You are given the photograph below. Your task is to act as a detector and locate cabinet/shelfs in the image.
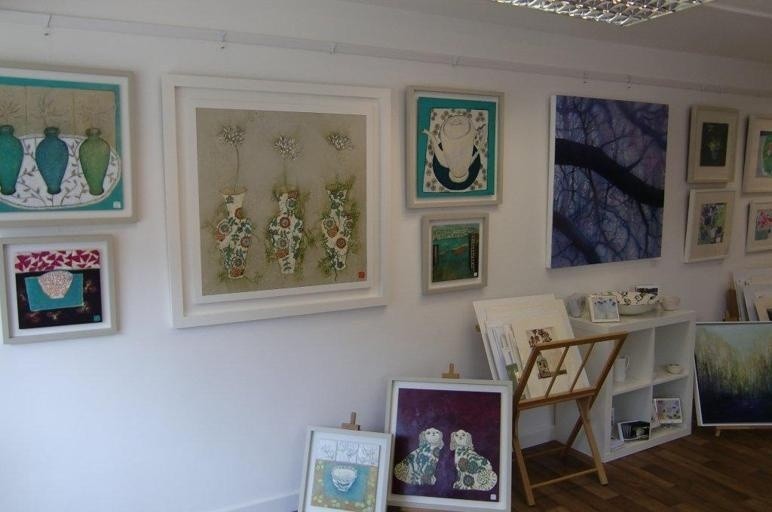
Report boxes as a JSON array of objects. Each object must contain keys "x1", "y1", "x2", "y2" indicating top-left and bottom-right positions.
[{"x1": 554, "y1": 310, "x2": 696, "y2": 463}]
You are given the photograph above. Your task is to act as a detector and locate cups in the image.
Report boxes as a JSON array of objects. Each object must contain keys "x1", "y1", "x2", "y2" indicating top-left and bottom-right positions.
[{"x1": 613, "y1": 354, "x2": 629, "y2": 382}]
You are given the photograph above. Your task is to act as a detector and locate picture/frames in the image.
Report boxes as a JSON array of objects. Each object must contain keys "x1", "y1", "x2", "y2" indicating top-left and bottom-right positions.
[
  {"x1": 420, "y1": 210, "x2": 489, "y2": 295},
  {"x1": 742, "y1": 113, "x2": 772, "y2": 194},
  {"x1": 694, "y1": 320, "x2": 772, "y2": 427},
  {"x1": 0, "y1": 65, "x2": 136, "y2": 226},
  {"x1": 683, "y1": 187, "x2": 737, "y2": 263},
  {"x1": 744, "y1": 198, "x2": 772, "y2": 254},
  {"x1": 297, "y1": 425, "x2": 394, "y2": 512},
  {"x1": 383, "y1": 376, "x2": 513, "y2": 512},
  {"x1": 588, "y1": 294, "x2": 620, "y2": 323},
  {"x1": 160, "y1": 72, "x2": 388, "y2": 328},
  {"x1": 405, "y1": 83, "x2": 506, "y2": 209},
  {"x1": 686, "y1": 102, "x2": 740, "y2": 185},
  {"x1": 0, "y1": 233, "x2": 119, "y2": 344},
  {"x1": 546, "y1": 90, "x2": 670, "y2": 267}
]
